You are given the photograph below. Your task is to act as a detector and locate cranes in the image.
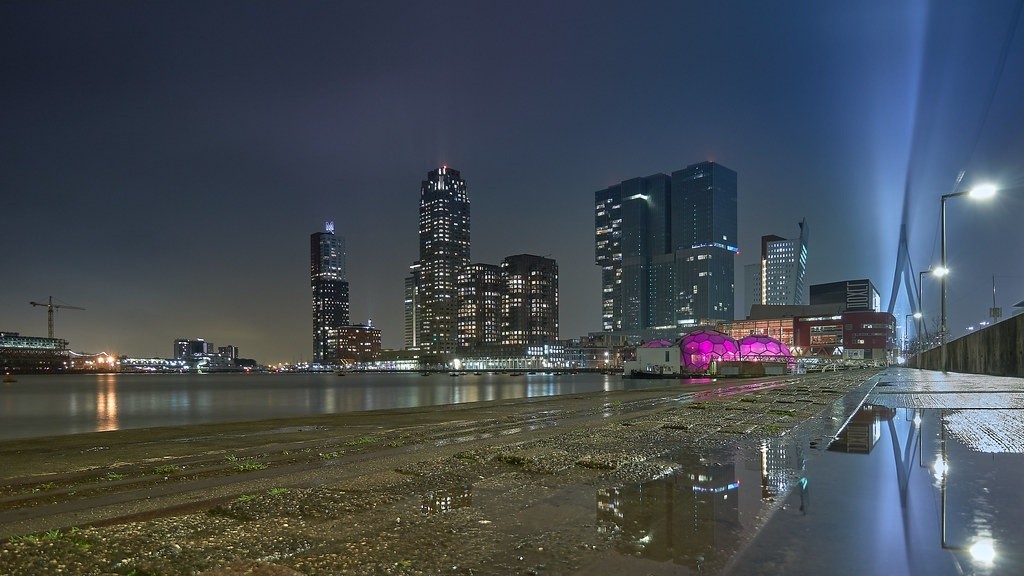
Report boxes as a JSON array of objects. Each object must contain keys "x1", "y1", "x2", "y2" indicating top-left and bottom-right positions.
[{"x1": 30, "y1": 296, "x2": 86, "y2": 338}]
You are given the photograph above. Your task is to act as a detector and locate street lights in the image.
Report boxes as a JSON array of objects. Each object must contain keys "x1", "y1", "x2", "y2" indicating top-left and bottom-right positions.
[
  {"x1": 905, "y1": 313, "x2": 922, "y2": 367},
  {"x1": 918, "y1": 267, "x2": 948, "y2": 369},
  {"x1": 941, "y1": 184, "x2": 997, "y2": 371},
  {"x1": 904, "y1": 408, "x2": 995, "y2": 565}
]
